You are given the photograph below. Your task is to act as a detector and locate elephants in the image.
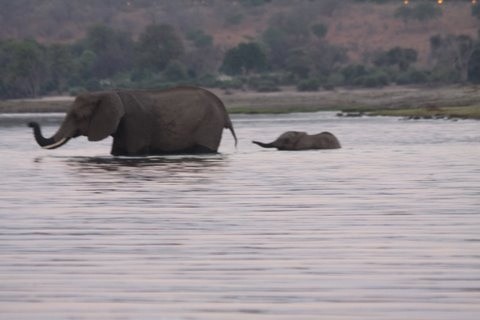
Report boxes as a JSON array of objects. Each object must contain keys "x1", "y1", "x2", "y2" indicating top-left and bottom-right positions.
[
  {"x1": 252, "y1": 131, "x2": 342, "y2": 151},
  {"x1": 27, "y1": 87, "x2": 238, "y2": 157}
]
